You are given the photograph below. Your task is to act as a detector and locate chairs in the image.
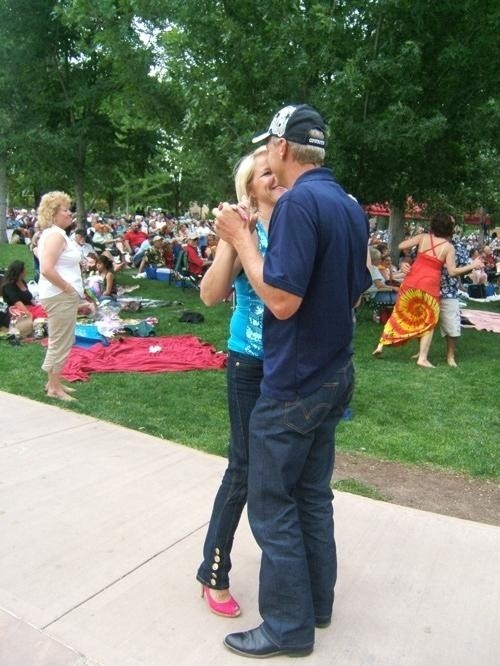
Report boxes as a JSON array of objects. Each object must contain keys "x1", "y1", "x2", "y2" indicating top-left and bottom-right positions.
[{"x1": 168, "y1": 240, "x2": 202, "y2": 294}]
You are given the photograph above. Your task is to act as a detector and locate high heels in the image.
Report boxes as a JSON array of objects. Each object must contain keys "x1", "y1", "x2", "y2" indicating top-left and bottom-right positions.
[{"x1": 197, "y1": 577, "x2": 244, "y2": 621}]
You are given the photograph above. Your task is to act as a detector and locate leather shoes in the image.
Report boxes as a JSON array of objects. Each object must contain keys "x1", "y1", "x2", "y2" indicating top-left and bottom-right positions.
[{"x1": 220, "y1": 619, "x2": 316, "y2": 660}]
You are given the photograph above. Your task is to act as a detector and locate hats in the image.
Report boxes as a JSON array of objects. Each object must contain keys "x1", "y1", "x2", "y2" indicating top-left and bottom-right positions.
[
  {"x1": 153, "y1": 230, "x2": 215, "y2": 241},
  {"x1": 248, "y1": 103, "x2": 327, "y2": 150}
]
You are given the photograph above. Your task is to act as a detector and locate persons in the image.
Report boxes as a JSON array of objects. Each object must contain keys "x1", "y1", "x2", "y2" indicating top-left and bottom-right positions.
[
  {"x1": 219, "y1": 104, "x2": 374, "y2": 659},
  {"x1": 194, "y1": 142, "x2": 358, "y2": 618},
  {"x1": 8, "y1": 196, "x2": 220, "y2": 317},
  {"x1": 3, "y1": 259, "x2": 35, "y2": 319},
  {"x1": 363, "y1": 213, "x2": 500, "y2": 317},
  {"x1": 36, "y1": 190, "x2": 86, "y2": 402},
  {"x1": 373, "y1": 211, "x2": 484, "y2": 370},
  {"x1": 401, "y1": 215, "x2": 481, "y2": 368}
]
